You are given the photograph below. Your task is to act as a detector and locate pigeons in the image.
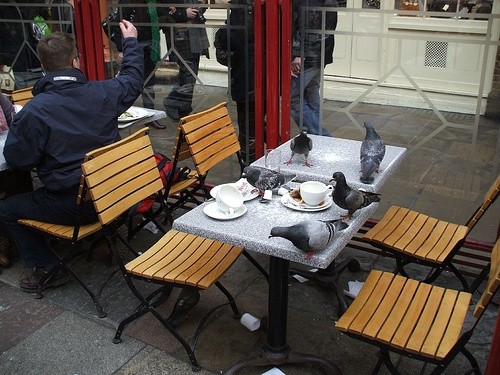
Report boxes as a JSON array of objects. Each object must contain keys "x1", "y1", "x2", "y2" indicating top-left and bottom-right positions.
[
  {"x1": 241, "y1": 165, "x2": 297, "y2": 197},
  {"x1": 358, "y1": 120, "x2": 386, "y2": 185},
  {"x1": 268, "y1": 217, "x2": 350, "y2": 261},
  {"x1": 328, "y1": 170, "x2": 383, "y2": 221},
  {"x1": 283, "y1": 126, "x2": 314, "y2": 169}
]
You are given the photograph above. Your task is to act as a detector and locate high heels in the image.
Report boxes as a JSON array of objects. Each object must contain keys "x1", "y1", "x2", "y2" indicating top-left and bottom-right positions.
[{"x1": 144, "y1": 120, "x2": 167, "y2": 129}]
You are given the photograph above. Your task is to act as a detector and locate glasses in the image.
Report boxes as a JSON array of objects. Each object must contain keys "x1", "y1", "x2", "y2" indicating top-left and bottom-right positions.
[{"x1": 74, "y1": 53, "x2": 82, "y2": 60}]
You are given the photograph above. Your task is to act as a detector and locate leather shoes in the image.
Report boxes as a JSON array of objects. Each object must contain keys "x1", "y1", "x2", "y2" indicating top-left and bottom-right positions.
[{"x1": 0, "y1": 235, "x2": 10, "y2": 268}]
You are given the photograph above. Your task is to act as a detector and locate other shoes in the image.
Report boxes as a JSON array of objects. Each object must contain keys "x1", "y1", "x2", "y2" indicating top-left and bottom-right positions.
[{"x1": 163, "y1": 101, "x2": 181, "y2": 122}]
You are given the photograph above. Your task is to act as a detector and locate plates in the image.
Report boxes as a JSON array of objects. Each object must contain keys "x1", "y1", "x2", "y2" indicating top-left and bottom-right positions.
[
  {"x1": 280, "y1": 192, "x2": 333, "y2": 212},
  {"x1": 210, "y1": 183, "x2": 260, "y2": 200},
  {"x1": 117, "y1": 106, "x2": 148, "y2": 122},
  {"x1": 203, "y1": 202, "x2": 247, "y2": 221}
]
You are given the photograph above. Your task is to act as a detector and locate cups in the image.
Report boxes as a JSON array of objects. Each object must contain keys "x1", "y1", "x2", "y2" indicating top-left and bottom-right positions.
[
  {"x1": 265, "y1": 149, "x2": 282, "y2": 172},
  {"x1": 216, "y1": 185, "x2": 246, "y2": 215},
  {"x1": 300, "y1": 181, "x2": 334, "y2": 206}
]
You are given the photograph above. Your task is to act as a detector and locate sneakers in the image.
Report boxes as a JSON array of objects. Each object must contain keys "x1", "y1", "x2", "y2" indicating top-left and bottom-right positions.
[{"x1": 19, "y1": 265, "x2": 69, "y2": 293}]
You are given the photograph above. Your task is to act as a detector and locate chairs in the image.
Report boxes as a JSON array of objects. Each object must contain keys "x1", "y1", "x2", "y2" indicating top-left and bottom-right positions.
[{"x1": 0, "y1": 87, "x2": 500, "y2": 375}]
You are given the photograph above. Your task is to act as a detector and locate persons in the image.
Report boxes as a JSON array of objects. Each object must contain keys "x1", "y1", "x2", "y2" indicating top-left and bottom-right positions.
[
  {"x1": 0, "y1": 19, "x2": 143, "y2": 292},
  {"x1": 212, "y1": 0, "x2": 281, "y2": 179},
  {"x1": 101, "y1": 0, "x2": 175, "y2": 129},
  {"x1": 161, "y1": 0, "x2": 209, "y2": 125},
  {"x1": 0, "y1": 0, "x2": 76, "y2": 73},
  {"x1": 0, "y1": 91, "x2": 18, "y2": 268},
  {"x1": 288, "y1": 1, "x2": 337, "y2": 137}
]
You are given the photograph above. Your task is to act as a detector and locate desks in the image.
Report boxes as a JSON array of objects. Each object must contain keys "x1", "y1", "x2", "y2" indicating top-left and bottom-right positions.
[
  {"x1": 173, "y1": 180, "x2": 380, "y2": 375},
  {"x1": 118, "y1": 106, "x2": 166, "y2": 145},
  {"x1": 250, "y1": 133, "x2": 407, "y2": 315}
]
